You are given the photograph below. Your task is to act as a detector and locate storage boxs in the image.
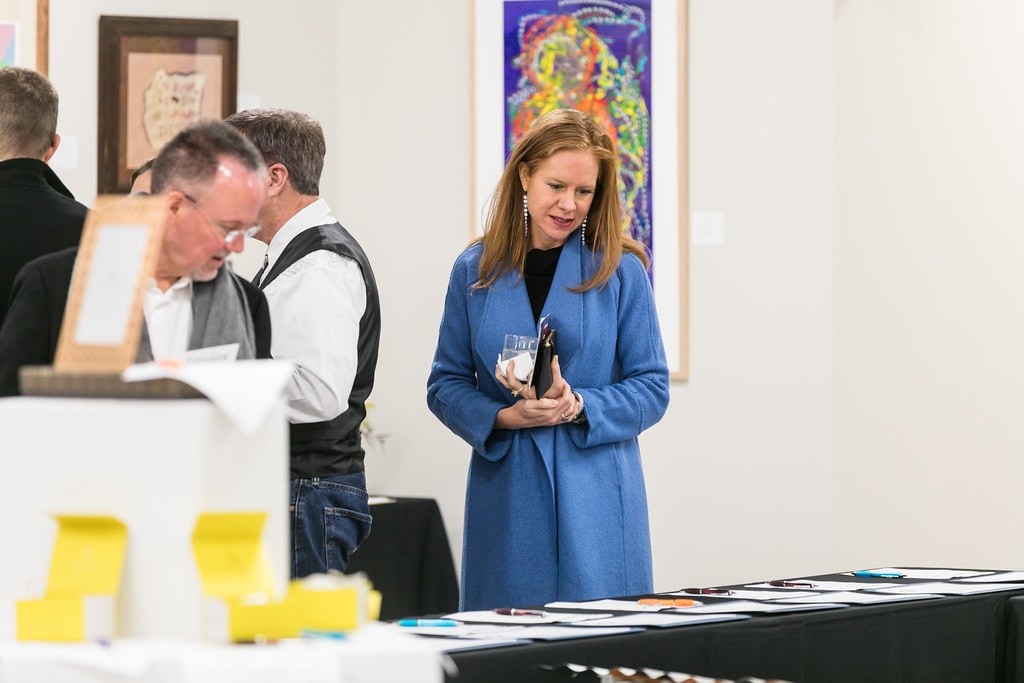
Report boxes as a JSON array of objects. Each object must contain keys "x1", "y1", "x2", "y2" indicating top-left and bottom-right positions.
[{"x1": 0, "y1": 397, "x2": 381, "y2": 647}]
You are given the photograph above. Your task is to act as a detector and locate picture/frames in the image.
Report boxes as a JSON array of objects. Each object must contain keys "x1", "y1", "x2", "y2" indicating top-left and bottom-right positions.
[
  {"x1": 468, "y1": 0, "x2": 690, "y2": 384},
  {"x1": 0, "y1": 0, "x2": 49, "y2": 77},
  {"x1": 97, "y1": 14, "x2": 238, "y2": 195}
]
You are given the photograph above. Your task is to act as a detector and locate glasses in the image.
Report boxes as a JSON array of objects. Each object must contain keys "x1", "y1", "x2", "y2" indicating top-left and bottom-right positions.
[{"x1": 182, "y1": 192, "x2": 261, "y2": 245}]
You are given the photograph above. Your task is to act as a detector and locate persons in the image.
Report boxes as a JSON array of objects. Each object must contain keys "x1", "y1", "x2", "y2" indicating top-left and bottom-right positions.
[
  {"x1": 224, "y1": 110, "x2": 380, "y2": 578},
  {"x1": 0, "y1": 119, "x2": 272, "y2": 399},
  {"x1": 0, "y1": 67, "x2": 89, "y2": 289},
  {"x1": 426, "y1": 109, "x2": 670, "y2": 611}
]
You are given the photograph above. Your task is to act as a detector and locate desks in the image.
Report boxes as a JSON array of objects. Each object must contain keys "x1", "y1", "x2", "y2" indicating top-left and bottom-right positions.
[
  {"x1": 346, "y1": 494, "x2": 459, "y2": 624},
  {"x1": 396, "y1": 564, "x2": 1024, "y2": 683},
  {"x1": 0, "y1": 621, "x2": 457, "y2": 683}
]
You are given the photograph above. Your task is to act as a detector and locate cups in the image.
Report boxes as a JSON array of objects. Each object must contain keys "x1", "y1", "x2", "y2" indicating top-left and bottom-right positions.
[{"x1": 502, "y1": 335, "x2": 538, "y2": 381}]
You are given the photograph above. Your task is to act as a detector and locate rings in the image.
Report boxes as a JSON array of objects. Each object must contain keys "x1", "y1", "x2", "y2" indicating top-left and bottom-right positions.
[
  {"x1": 562, "y1": 414, "x2": 570, "y2": 420},
  {"x1": 511, "y1": 384, "x2": 524, "y2": 397}
]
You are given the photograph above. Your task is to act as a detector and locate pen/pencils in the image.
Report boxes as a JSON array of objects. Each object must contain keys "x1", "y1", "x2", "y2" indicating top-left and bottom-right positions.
[
  {"x1": 497, "y1": 607, "x2": 549, "y2": 618},
  {"x1": 399, "y1": 619, "x2": 463, "y2": 627},
  {"x1": 639, "y1": 598, "x2": 702, "y2": 607},
  {"x1": 851, "y1": 571, "x2": 908, "y2": 578},
  {"x1": 770, "y1": 580, "x2": 813, "y2": 588},
  {"x1": 685, "y1": 588, "x2": 734, "y2": 595}
]
